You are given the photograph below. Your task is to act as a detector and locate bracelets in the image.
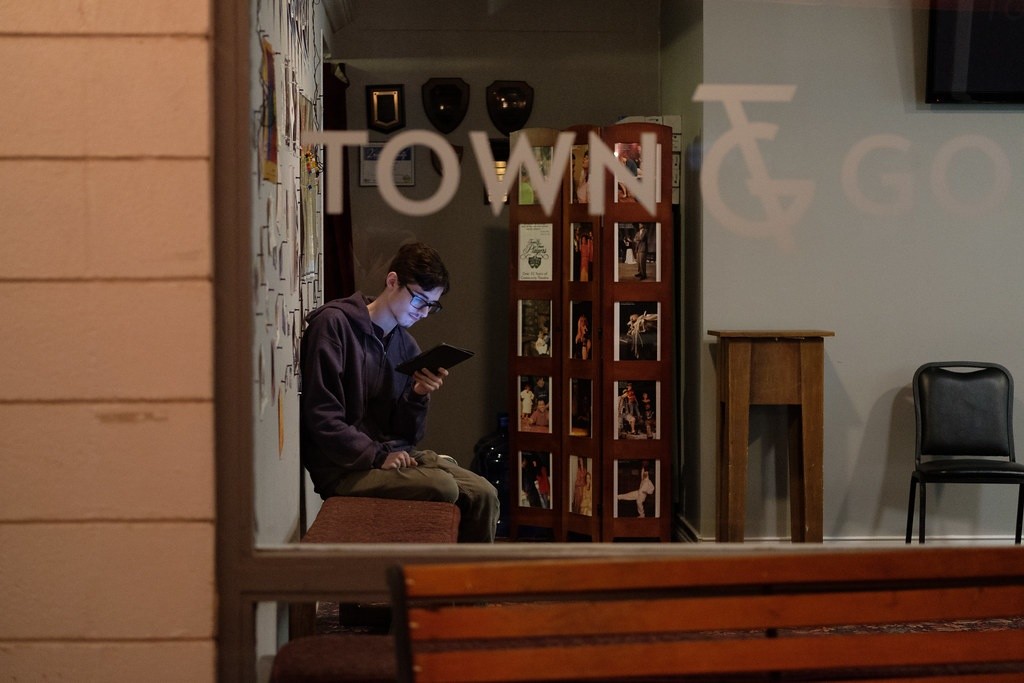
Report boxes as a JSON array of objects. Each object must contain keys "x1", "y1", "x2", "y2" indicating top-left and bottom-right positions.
[{"x1": 582, "y1": 345, "x2": 586, "y2": 347}]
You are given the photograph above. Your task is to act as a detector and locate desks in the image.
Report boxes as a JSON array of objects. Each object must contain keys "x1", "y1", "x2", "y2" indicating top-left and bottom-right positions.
[{"x1": 707, "y1": 330, "x2": 835, "y2": 544}]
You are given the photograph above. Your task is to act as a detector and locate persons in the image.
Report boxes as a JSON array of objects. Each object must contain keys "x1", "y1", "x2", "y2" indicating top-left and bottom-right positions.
[
  {"x1": 573, "y1": 225, "x2": 596, "y2": 281},
  {"x1": 521, "y1": 143, "x2": 646, "y2": 203},
  {"x1": 526, "y1": 310, "x2": 657, "y2": 360},
  {"x1": 299, "y1": 243, "x2": 502, "y2": 607},
  {"x1": 623, "y1": 222, "x2": 649, "y2": 281},
  {"x1": 521, "y1": 376, "x2": 549, "y2": 426},
  {"x1": 520, "y1": 452, "x2": 656, "y2": 519},
  {"x1": 617, "y1": 383, "x2": 654, "y2": 439}
]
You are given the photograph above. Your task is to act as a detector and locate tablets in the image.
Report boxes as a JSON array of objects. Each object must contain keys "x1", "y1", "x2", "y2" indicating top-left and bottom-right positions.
[{"x1": 394, "y1": 343, "x2": 476, "y2": 377}]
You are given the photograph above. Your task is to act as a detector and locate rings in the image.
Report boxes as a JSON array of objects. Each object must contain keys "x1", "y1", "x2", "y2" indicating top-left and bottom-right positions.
[{"x1": 428, "y1": 381, "x2": 433, "y2": 384}]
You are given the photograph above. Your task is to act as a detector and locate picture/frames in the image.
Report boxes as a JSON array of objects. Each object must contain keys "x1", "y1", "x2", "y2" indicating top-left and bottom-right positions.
[{"x1": 365, "y1": 84, "x2": 406, "y2": 134}]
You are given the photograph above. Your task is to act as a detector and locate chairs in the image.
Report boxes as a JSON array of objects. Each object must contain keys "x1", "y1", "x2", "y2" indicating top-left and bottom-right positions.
[{"x1": 905, "y1": 361, "x2": 1024, "y2": 544}]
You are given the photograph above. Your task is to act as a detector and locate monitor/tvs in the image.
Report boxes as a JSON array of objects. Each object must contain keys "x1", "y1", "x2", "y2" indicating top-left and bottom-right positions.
[{"x1": 925, "y1": 0, "x2": 1024, "y2": 110}]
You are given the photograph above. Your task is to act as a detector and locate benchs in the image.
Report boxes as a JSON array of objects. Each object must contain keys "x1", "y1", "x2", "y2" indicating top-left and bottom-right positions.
[
  {"x1": 286, "y1": 496, "x2": 461, "y2": 638},
  {"x1": 404, "y1": 545, "x2": 1024, "y2": 683}
]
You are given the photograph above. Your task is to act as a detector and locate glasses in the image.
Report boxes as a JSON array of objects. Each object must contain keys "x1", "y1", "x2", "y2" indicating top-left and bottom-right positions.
[{"x1": 404, "y1": 283, "x2": 443, "y2": 314}]
